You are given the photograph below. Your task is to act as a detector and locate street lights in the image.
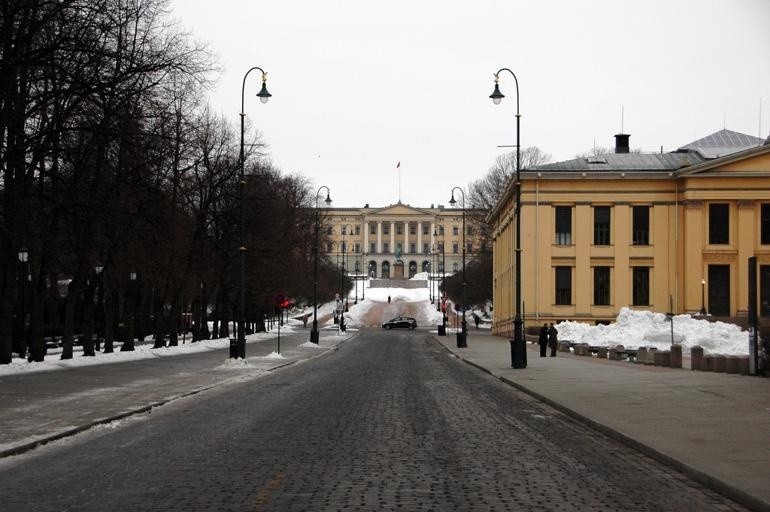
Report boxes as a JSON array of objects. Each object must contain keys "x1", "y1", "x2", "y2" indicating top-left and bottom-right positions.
[
  {"x1": 229, "y1": 67, "x2": 272, "y2": 361},
  {"x1": 449, "y1": 187, "x2": 467, "y2": 348},
  {"x1": 310, "y1": 185, "x2": 332, "y2": 345},
  {"x1": 429, "y1": 224, "x2": 447, "y2": 336},
  {"x1": 340, "y1": 223, "x2": 364, "y2": 336},
  {"x1": 490, "y1": 68, "x2": 527, "y2": 370}
]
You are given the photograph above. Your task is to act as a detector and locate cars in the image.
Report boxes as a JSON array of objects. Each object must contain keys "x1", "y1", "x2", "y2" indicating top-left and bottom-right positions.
[{"x1": 380, "y1": 316, "x2": 417, "y2": 330}]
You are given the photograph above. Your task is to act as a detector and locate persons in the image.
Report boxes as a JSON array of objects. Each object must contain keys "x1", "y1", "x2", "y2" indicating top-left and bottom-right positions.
[
  {"x1": 538, "y1": 323, "x2": 549, "y2": 357},
  {"x1": 388, "y1": 296, "x2": 391, "y2": 304},
  {"x1": 548, "y1": 322, "x2": 558, "y2": 357}
]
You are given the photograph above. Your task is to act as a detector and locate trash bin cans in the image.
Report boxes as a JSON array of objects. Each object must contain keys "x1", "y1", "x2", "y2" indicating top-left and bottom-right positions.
[
  {"x1": 457, "y1": 333, "x2": 466, "y2": 347},
  {"x1": 230, "y1": 339, "x2": 245, "y2": 358},
  {"x1": 438, "y1": 325, "x2": 445, "y2": 335},
  {"x1": 511, "y1": 340, "x2": 528, "y2": 368}
]
